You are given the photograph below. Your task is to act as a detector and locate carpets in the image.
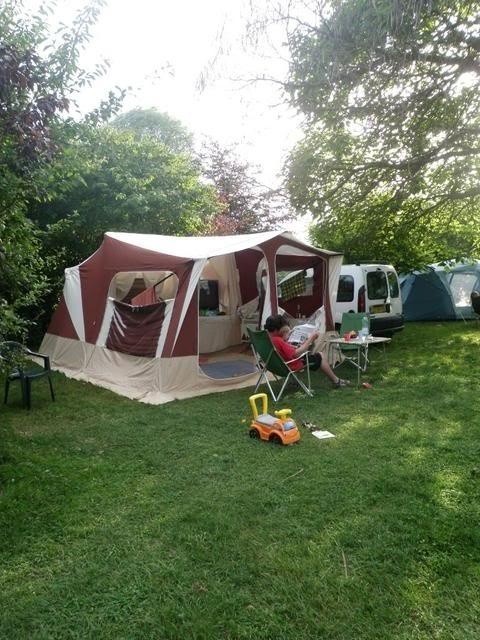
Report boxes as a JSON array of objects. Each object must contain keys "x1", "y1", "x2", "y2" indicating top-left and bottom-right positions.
[{"x1": 200, "y1": 353, "x2": 255, "y2": 379}]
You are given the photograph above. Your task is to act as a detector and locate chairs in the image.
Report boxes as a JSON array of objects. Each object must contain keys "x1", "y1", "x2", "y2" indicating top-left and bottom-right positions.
[
  {"x1": 1, "y1": 341, "x2": 56, "y2": 411},
  {"x1": 246, "y1": 313, "x2": 370, "y2": 401}
]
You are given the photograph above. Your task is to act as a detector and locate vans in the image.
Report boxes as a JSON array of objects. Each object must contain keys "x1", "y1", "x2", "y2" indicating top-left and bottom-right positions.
[{"x1": 279, "y1": 264, "x2": 406, "y2": 337}]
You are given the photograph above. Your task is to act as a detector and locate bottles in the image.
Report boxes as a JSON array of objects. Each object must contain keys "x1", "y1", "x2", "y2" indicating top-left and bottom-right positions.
[{"x1": 362, "y1": 316, "x2": 369, "y2": 338}]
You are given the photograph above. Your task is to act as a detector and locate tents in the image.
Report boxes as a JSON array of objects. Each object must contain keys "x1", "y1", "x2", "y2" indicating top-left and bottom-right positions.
[
  {"x1": 395, "y1": 255, "x2": 480, "y2": 322},
  {"x1": 23, "y1": 226, "x2": 345, "y2": 407}
]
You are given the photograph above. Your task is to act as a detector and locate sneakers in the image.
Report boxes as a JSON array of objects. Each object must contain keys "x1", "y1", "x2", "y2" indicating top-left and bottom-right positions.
[{"x1": 333, "y1": 378, "x2": 351, "y2": 388}]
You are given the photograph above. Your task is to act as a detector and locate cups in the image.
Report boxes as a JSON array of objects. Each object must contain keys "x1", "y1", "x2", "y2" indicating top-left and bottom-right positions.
[{"x1": 344, "y1": 334, "x2": 350, "y2": 341}]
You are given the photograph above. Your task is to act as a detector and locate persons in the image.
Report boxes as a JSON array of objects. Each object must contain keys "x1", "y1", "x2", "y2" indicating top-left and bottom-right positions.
[
  {"x1": 262, "y1": 312, "x2": 352, "y2": 390},
  {"x1": 470, "y1": 290, "x2": 480, "y2": 314},
  {"x1": 280, "y1": 325, "x2": 291, "y2": 340}
]
